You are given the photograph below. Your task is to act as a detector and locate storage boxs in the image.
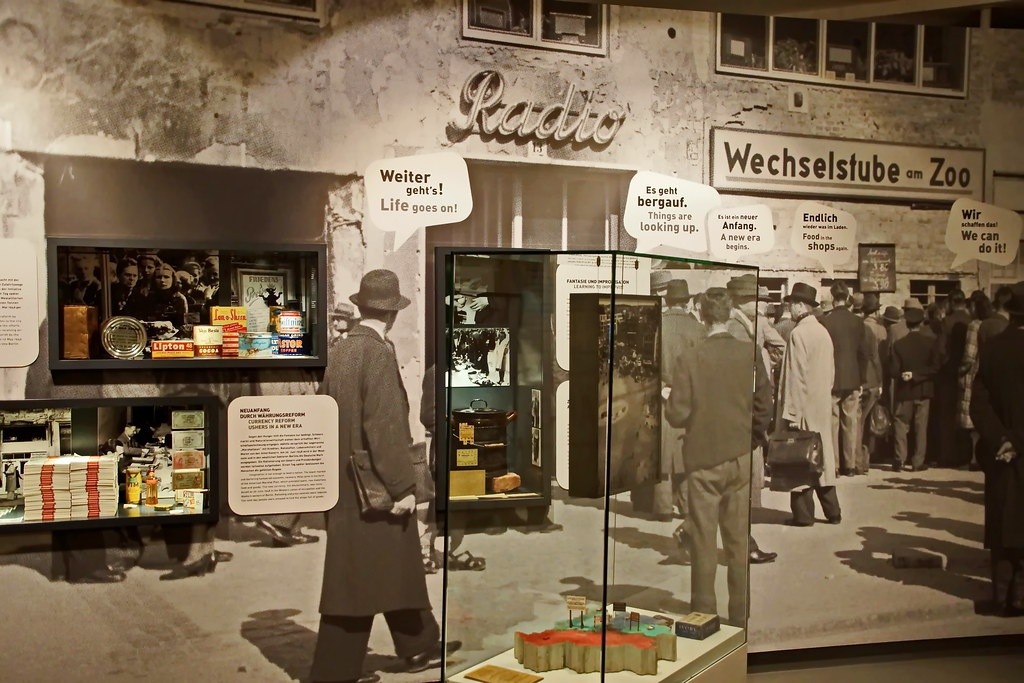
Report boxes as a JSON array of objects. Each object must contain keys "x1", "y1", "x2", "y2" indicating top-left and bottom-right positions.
[
  {"x1": 211, "y1": 306, "x2": 248, "y2": 357},
  {"x1": 64, "y1": 306, "x2": 98, "y2": 360},
  {"x1": 193, "y1": 325, "x2": 223, "y2": 357},
  {"x1": 152, "y1": 341, "x2": 194, "y2": 358}
]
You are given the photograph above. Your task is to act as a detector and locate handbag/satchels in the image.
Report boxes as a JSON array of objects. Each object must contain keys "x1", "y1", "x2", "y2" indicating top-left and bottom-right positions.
[
  {"x1": 869, "y1": 405, "x2": 894, "y2": 438},
  {"x1": 767, "y1": 428, "x2": 825, "y2": 477},
  {"x1": 351, "y1": 442, "x2": 435, "y2": 513}
]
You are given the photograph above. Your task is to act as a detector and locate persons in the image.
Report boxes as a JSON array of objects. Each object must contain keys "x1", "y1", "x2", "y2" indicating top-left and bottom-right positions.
[
  {"x1": 66, "y1": 252, "x2": 220, "y2": 337},
  {"x1": 443, "y1": 292, "x2": 493, "y2": 312},
  {"x1": 630, "y1": 272, "x2": 1023, "y2": 619},
  {"x1": 309, "y1": 270, "x2": 486, "y2": 683}
]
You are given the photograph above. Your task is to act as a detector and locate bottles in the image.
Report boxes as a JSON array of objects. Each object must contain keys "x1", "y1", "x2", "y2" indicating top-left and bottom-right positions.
[{"x1": 145, "y1": 465, "x2": 159, "y2": 507}]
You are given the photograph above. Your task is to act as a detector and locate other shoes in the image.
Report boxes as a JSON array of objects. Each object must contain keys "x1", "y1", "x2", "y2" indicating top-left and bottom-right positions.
[
  {"x1": 974, "y1": 600, "x2": 1024, "y2": 617},
  {"x1": 256, "y1": 524, "x2": 320, "y2": 546}
]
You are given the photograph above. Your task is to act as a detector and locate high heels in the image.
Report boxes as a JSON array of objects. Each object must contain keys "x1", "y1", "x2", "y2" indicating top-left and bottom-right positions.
[{"x1": 159, "y1": 550, "x2": 218, "y2": 580}]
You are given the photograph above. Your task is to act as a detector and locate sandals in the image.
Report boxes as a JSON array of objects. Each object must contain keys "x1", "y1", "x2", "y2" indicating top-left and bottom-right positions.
[
  {"x1": 447, "y1": 550, "x2": 486, "y2": 572},
  {"x1": 420, "y1": 543, "x2": 439, "y2": 573}
]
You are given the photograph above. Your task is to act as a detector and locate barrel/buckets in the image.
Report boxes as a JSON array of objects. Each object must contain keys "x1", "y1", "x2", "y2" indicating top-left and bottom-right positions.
[
  {"x1": 238, "y1": 331, "x2": 272, "y2": 358},
  {"x1": 279, "y1": 311, "x2": 304, "y2": 356},
  {"x1": 451, "y1": 426, "x2": 507, "y2": 494}
]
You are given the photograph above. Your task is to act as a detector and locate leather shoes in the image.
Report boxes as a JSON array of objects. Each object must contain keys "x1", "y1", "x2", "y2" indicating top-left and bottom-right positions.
[
  {"x1": 167, "y1": 551, "x2": 233, "y2": 564},
  {"x1": 355, "y1": 674, "x2": 380, "y2": 683},
  {"x1": 785, "y1": 517, "x2": 812, "y2": 527},
  {"x1": 53, "y1": 568, "x2": 127, "y2": 584},
  {"x1": 750, "y1": 550, "x2": 777, "y2": 564},
  {"x1": 836, "y1": 468, "x2": 869, "y2": 479},
  {"x1": 830, "y1": 516, "x2": 842, "y2": 523},
  {"x1": 893, "y1": 463, "x2": 905, "y2": 471},
  {"x1": 673, "y1": 525, "x2": 690, "y2": 562},
  {"x1": 407, "y1": 641, "x2": 462, "y2": 672},
  {"x1": 913, "y1": 464, "x2": 929, "y2": 471}
]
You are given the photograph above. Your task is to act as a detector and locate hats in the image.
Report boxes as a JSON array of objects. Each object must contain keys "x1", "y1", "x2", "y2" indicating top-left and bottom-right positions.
[
  {"x1": 329, "y1": 303, "x2": 355, "y2": 320},
  {"x1": 727, "y1": 274, "x2": 773, "y2": 302},
  {"x1": 349, "y1": 270, "x2": 410, "y2": 310},
  {"x1": 814, "y1": 299, "x2": 834, "y2": 317},
  {"x1": 785, "y1": 283, "x2": 820, "y2": 307},
  {"x1": 650, "y1": 271, "x2": 696, "y2": 298},
  {"x1": 767, "y1": 304, "x2": 788, "y2": 317},
  {"x1": 846, "y1": 293, "x2": 925, "y2": 324},
  {"x1": 1003, "y1": 281, "x2": 1024, "y2": 316}
]
